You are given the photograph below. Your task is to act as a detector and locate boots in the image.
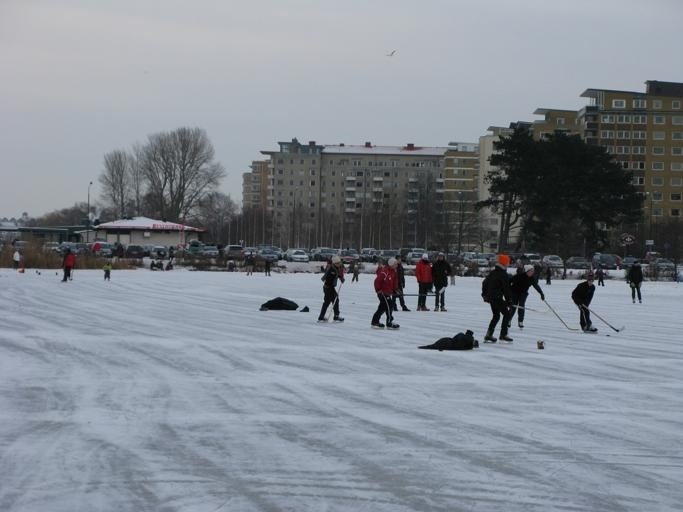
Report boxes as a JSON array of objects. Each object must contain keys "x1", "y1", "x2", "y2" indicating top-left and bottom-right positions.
[
  {"x1": 500, "y1": 328, "x2": 513, "y2": 341},
  {"x1": 484, "y1": 328, "x2": 497, "y2": 341}
]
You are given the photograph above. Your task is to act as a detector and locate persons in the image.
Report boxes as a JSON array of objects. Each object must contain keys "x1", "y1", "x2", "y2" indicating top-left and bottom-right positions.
[
  {"x1": 19, "y1": 247, "x2": 26, "y2": 268},
  {"x1": 92, "y1": 240, "x2": 102, "y2": 257},
  {"x1": 244, "y1": 252, "x2": 271, "y2": 277},
  {"x1": 59, "y1": 246, "x2": 75, "y2": 282},
  {"x1": 60, "y1": 246, "x2": 68, "y2": 267},
  {"x1": 164, "y1": 261, "x2": 172, "y2": 271},
  {"x1": 101, "y1": 259, "x2": 112, "y2": 281},
  {"x1": 481, "y1": 255, "x2": 550, "y2": 344},
  {"x1": 117, "y1": 242, "x2": 124, "y2": 260},
  {"x1": 369, "y1": 252, "x2": 456, "y2": 328},
  {"x1": 562, "y1": 260, "x2": 643, "y2": 333},
  {"x1": 12, "y1": 248, "x2": 20, "y2": 269},
  {"x1": 167, "y1": 244, "x2": 175, "y2": 260},
  {"x1": 148, "y1": 259, "x2": 157, "y2": 270},
  {"x1": 155, "y1": 258, "x2": 164, "y2": 270},
  {"x1": 315, "y1": 255, "x2": 360, "y2": 321}
]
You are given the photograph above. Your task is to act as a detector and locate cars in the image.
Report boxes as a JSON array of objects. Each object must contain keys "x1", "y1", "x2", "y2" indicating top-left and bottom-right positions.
[
  {"x1": 149, "y1": 246, "x2": 168, "y2": 259},
  {"x1": 217, "y1": 244, "x2": 360, "y2": 265},
  {"x1": 110, "y1": 242, "x2": 123, "y2": 256},
  {"x1": 0, "y1": 238, "x2": 6, "y2": 252},
  {"x1": 203, "y1": 246, "x2": 218, "y2": 257},
  {"x1": 359, "y1": 247, "x2": 496, "y2": 267},
  {"x1": 512, "y1": 250, "x2": 677, "y2": 273},
  {"x1": 56, "y1": 242, "x2": 72, "y2": 253},
  {"x1": 125, "y1": 244, "x2": 147, "y2": 257}
]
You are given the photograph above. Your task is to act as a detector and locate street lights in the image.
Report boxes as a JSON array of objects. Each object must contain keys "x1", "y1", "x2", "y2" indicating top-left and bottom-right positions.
[
  {"x1": 456, "y1": 191, "x2": 463, "y2": 247},
  {"x1": 86, "y1": 181, "x2": 93, "y2": 241},
  {"x1": 238, "y1": 240, "x2": 243, "y2": 247}
]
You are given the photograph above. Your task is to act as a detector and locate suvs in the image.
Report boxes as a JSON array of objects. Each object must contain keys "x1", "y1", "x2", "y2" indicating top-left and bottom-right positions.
[
  {"x1": 183, "y1": 243, "x2": 202, "y2": 257},
  {"x1": 69, "y1": 243, "x2": 89, "y2": 254},
  {"x1": 41, "y1": 241, "x2": 59, "y2": 255},
  {"x1": 87, "y1": 242, "x2": 113, "y2": 258},
  {"x1": 11, "y1": 240, "x2": 29, "y2": 255}
]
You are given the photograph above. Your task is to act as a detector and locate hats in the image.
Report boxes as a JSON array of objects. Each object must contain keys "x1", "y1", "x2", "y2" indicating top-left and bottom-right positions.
[
  {"x1": 588, "y1": 275, "x2": 594, "y2": 280},
  {"x1": 331, "y1": 255, "x2": 340, "y2": 264},
  {"x1": 499, "y1": 255, "x2": 510, "y2": 266},
  {"x1": 387, "y1": 258, "x2": 398, "y2": 267},
  {"x1": 523, "y1": 264, "x2": 534, "y2": 273},
  {"x1": 421, "y1": 254, "x2": 428, "y2": 259}
]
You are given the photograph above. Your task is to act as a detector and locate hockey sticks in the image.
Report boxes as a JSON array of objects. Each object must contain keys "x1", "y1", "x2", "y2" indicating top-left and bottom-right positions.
[
  {"x1": 513, "y1": 305, "x2": 551, "y2": 312},
  {"x1": 398, "y1": 286, "x2": 446, "y2": 296},
  {"x1": 582, "y1": 303, "x2": 625, "y2": 332},
  {"x1": 544, "y1": 299, "x2": 579, "y2": 331}
]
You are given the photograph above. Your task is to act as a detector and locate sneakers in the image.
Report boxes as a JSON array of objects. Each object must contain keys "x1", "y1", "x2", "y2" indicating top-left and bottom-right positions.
[
  {"x1": 393, "y1": 306, "x2": 447, "y2": 311},
  {"x1": 588, "y1": 326, "x2": 597, "y2": 331},
  {"x1": 518, "y1": 322, "x2": 524, "y2": 327},
  {"x1": 583, "y1": 325, "x2": 587, "y2": 331},
  {"x1": 334, "y1": 315, "x2": 344, "y2": 321},
  {"x1": 387, "y1": 323, "x2": 399, "y2": 328},
  {"x1": 507, "y1": 321, "x2": 510, "y2": 327},
  {"x1": 372, "y1": 322, "x2": 384, "y2": 327}
]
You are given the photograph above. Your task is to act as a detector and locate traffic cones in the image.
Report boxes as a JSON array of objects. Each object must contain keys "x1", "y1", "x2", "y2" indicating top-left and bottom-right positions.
[{"x1": 17, "y1": 269, "x2": 24, "y2": 273}]
